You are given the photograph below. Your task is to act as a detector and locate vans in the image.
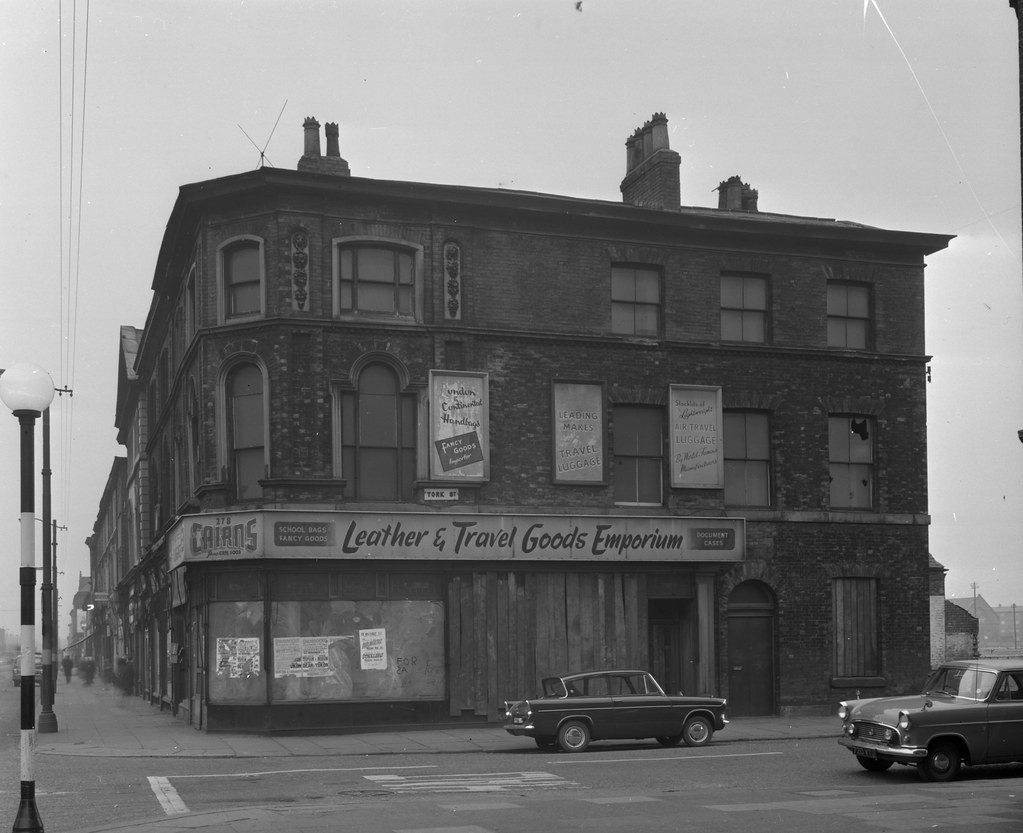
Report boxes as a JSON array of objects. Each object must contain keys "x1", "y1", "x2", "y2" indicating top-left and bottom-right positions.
[{"x1": 14, "y1": 655, "x2": 43, "y2": 686}]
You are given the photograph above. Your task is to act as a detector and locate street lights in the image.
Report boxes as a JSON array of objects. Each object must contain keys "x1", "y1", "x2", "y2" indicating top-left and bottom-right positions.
[{"x1": 0, "y1": 358, "x2": 57, "y2": 833}]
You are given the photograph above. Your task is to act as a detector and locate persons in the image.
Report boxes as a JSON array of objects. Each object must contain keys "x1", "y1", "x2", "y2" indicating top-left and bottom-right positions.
[{"x1": 62, "y1": 654, "x2": 73, "y2": 684}]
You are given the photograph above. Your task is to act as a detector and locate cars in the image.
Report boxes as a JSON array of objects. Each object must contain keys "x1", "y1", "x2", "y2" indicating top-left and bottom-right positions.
[
  {"x1": 837, "y1": 660, "x2": 1023, "y2": 781},
  {"x1": 501, "y1": 670, "x2": 729, "y2": 752}
]
